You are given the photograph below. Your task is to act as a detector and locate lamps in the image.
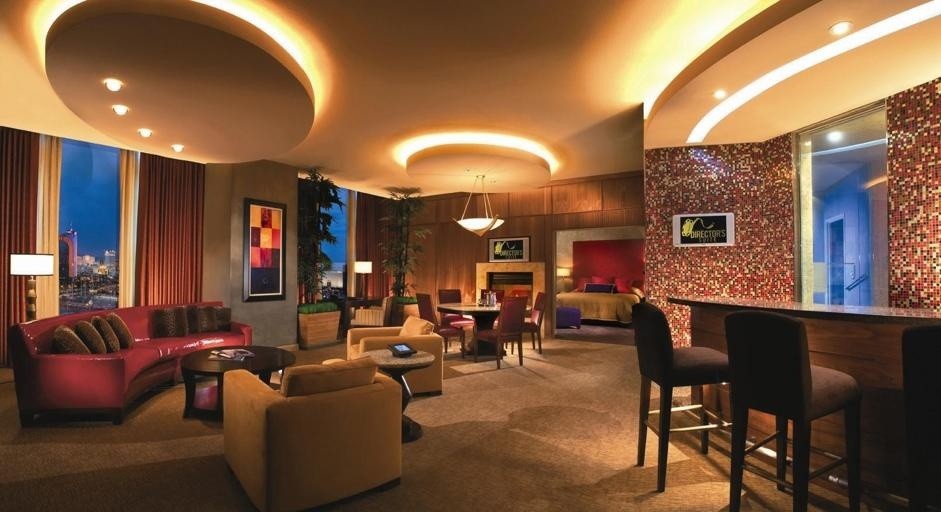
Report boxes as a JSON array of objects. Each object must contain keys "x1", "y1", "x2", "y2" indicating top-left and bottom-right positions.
[
  {"x1": 9, "y1": 252, "x2": 54, "y2": 320},
  {"x1": 354, "y1": 261, "x2": 373, "y2": 301},
  {"x1": 455, "y1": 174, "x2": 505, "y2": 237},
  {"x1": 557, "y1": 267, "x2": 571, "y2": 280}
]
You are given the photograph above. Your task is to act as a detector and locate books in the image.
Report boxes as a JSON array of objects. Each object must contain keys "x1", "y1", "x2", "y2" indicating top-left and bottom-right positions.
[{"x1": 208, "y1": 348, "x2": 255, "y2": 362}]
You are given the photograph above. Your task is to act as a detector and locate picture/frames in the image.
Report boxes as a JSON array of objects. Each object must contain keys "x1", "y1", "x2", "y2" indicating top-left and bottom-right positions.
[
  {"x1": 488, "y1": 236, "x2": 531, "y2": 262},
  {"x1": 243, "y1": 196, "x2": 287, "y2": 303}
]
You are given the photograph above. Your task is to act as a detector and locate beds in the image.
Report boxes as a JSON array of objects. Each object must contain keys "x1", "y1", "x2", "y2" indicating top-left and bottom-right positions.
[{"x1": 556, "y1": 290, "x2": 639, "y2": 324}]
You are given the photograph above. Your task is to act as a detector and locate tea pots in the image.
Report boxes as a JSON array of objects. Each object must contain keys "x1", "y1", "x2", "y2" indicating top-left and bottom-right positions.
[{"x1": 483, "y1": 291, "x2": 495, "y2": 306}]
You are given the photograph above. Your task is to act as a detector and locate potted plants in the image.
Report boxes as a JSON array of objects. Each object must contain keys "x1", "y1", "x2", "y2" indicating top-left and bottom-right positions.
[
  {"x1": 378, "y1": 296, "x2": 420, "y2": 325},
  {"x1": 299, "y1": 301, "x2": 343, "y2": 350}
]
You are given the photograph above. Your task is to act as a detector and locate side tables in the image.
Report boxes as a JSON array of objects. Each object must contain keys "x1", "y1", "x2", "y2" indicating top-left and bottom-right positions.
[
  {"x1": 360, "y1": 347, "x2": 436, "y2": 445},
  {"x1": 348, "y1": 296, "x2": 373, "y2": 318}
]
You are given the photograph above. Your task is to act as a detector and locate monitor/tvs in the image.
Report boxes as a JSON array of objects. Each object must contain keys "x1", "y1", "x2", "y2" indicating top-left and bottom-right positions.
[{"x1": 387, "y1": 343, "x2": 416, "y2": 355}]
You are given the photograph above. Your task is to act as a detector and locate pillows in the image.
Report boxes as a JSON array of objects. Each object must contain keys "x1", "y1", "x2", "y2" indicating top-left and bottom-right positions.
[
  {"x1": 51, "y1": 310, "x2": 134, "y2": 355},
  {"x1": 399, "y1": 315, "x2": 435, "y2": 337},
  {"x1": 280, "y1": 355, "x2": 378, "y2": 397},
  {"x1": 577, "y1": 275, "x2": 644, "y2": 294},
  {"x1": 160, "y1": 304, "x2": 232, "y2": 335}
]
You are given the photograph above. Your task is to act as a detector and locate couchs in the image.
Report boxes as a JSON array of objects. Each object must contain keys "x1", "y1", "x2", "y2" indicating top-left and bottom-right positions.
[{"x1": 9, "y1": 300, "x2": 252, "y2": 427}]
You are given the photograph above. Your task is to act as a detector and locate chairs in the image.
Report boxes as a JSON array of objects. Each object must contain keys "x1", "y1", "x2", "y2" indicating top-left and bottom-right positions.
[
  {"x1": 902, "y1": 323, "x2": 941, "y2": 511},
  {"x1": 350, "y1": 297, "x2": 388, "y2": 327},
  {"x1": 416, "y1": 287, "x2": 547, "y2": 369},
  {"x1": 221, "y1": 367, "x2": 402, "y2": 512},
  {"x1": 630, "y1": 301, "x2": 730, "y2": 492},
  {"x1": 724, "y1": 311, "x2": 862, "y2": 512},
  {"x1": 346, "y1": 325, "x2": 443, "y2": 394}
]
